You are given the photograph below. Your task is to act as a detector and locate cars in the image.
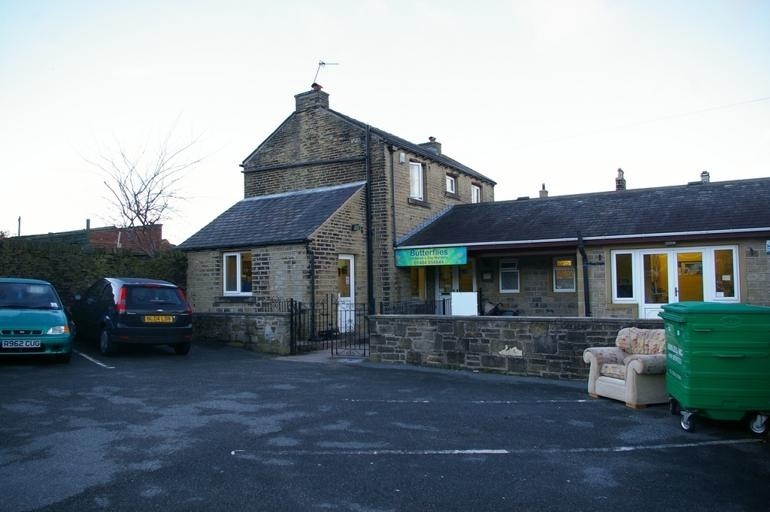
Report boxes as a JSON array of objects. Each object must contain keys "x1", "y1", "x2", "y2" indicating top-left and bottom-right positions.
[
  {"x1": 68, "y1": 275, "x2": 195, "y2": 356},
  {"x1": 0, "y1": 276, "x2": 76, "y2": 365}
]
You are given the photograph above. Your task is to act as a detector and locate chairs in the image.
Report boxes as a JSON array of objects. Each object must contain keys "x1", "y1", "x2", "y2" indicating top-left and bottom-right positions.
[{"x1": 582, "y1": 326, "x2": 669, "y2": 410}]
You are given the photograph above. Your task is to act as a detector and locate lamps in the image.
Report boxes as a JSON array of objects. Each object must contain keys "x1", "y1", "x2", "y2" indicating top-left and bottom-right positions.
[{"x1": 351, "y1": 224, "x2": 361, "y2": 232}]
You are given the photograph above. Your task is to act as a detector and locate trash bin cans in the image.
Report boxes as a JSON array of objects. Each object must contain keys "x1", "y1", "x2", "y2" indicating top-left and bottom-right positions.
[{"x1": 659, "y1": 302, "x2": 769, "y2": 435}]
